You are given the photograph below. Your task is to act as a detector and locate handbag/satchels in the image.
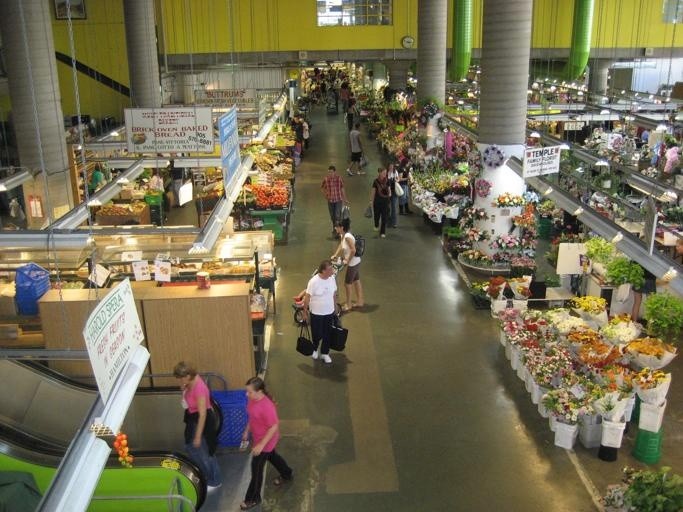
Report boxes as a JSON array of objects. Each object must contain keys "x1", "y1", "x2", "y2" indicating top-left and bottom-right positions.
[
  {"x1": 395, "y1": 170, "x2": 404, "y2": 197},
  {"x1": 297, "y1": 320, "x2": 314, "y2": 355},
  {"x1": 341, "y1": 202, "x2": 350, "y2": 220},
  {"x1": 364, "y1": 201, "x2": 373, "y2": 218},
  {"x1": 330, "y1": 313, "x2": 348, "y2": 352},
  {"x1": 359, "y1": 151, "x2": 370, "y2": 168}
]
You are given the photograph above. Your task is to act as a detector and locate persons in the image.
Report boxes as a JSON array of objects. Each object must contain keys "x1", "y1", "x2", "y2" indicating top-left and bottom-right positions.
[
  {"x1": 347, "y1": 123, "x2": 367, "y2": 176},
  {"x1": 330, "y1": 217, "x2": 366, "y2": 311},
  {"x1": 239, "y1": 377, "x2": 293, "y2": 510},
  {"x1": 90, "y1": 162, "x2": 104, "y2": 189},
  {"x1": 148, "y1": 168, "x2": 167, "y2": 221},
  {"x1": 329, "y1": 67, "x2": 334, "y2": 78},
  {"x1": 346, "y1": 78, "x2": 352, "y2": 93},
  {"x1": 314, "y1": 68, "x2": 320, "y2": 76},
  {"x1": 173, "y1": 361, "x2": 224, "y2": 492},
  {"x1": 315, "y1": 82, "x2": 326, "y2": 106},
  {"x1": 338, "y1": 70, "x2": 342, "y2": 78},
  {"x1": 340, "y1": 82, "x2": 349, "y2": 113},
  {"x1": 320, "y1": 70, "x2": 325, "y2": 78},
  {"x1": 320, "y1": 165, "x2": 346, "y2": 240},
  {"x1": 7, "y1": 190, "x2": 25, "y2": 219},
  {"x1": 302, "y1": 260, "x2": 338, "y2": 364},
  {"x1": 293, "y1": 116, "x2": 304, "y2": 158},
  {"x1": 299, "y1": 118, "x2": 309, "y2": 149},
  {"x1": 345, "y1": 98, "x2": 357, "y2": 131},
  {"x1": 386, "y1": 161, "x2": 399, "y2": 228},
  {"x1": 321, "y1": 81, "x2": 326, "y2": 100},
  {"x1": 397, "y1": 158, "x2": 414, "y2": 215},
  {"x1": 370, "y1": 167, "x2": 392, "y2": 238}
]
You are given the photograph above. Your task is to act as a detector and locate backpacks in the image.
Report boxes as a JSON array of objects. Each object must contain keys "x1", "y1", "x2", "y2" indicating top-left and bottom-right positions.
[{"x1": 353, "y1": 235, "x2": 366, "y2": 257}]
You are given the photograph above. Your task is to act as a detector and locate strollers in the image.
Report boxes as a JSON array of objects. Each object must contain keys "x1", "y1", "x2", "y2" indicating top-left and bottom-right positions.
[{"x1": 292, "y1": 257, "x2": 346, "y2": 323}]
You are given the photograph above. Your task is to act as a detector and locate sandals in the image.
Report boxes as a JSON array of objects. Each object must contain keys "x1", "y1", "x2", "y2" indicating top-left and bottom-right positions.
[
  {"x1": 274, "y1": 474, "x2": 293, "y2": 484},
  {"x1": 351, "y1": 302, "x2": 364, "y2": 308},
  {"x1": 240, "y1": 497, "x2": 262, "y2": 508},
  {"x1": 341, "y1": 304, "x2": 352, "y2": 311}
]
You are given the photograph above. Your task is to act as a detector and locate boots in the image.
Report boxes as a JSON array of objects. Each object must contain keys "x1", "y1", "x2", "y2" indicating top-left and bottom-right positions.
[
  {"x1": 404, "y1": 204, "x2": 414, "y2": 214},
  {"x1": 399, "y1": 205, "x2": 408, "y2": 215}
]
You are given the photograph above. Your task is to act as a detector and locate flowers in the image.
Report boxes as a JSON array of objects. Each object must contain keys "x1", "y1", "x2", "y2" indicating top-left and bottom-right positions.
[
  {"x1": 497, "y1": 192, "x2": 513, "y2": 208},
  {"x1": 622, "y1": 338, "x2": 674, "y2": 359},
  {"x1": 603, "y1": 313, "x2": 636, "y2": 342},
  {"x1": 636, "y1": 367, "x2": 666, "y2": 389},
  {"x1": 477, "y1": 180, "x2": 491, "y2": 198},
  {"x1": 483, "y1": 145, "x2": 505, "y2": 168},
  {"x1": 493, "y1": 235, "x2": 521, "y2": 251},
  {"x1": 570, "y1": 296, "x2": 591, "y2": 308},
  {"x1": 583, "y1": 298, "x2": 607, "y2": 314},
  {"x1": 508, "y1": 215, "x2": 525, "y2": 235}
]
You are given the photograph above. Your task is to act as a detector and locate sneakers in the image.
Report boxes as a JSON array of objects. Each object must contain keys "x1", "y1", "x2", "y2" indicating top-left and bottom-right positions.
[
  {"x1": 347, "y1": 169, "x2": 353, "y2": 176},
  {"x1": 374, "y1": 227, "x2": 378, "y2": 231},
  {"x1": 311, "y1": 350, "x2": 319, "y2": 360},
  {"x1": 380, "y1": 233, "x2": 385, "y2": 238},
  {"x1": 206, "y1": 482, "x2": 223, "y2": 491},
  {"x1": 320, "y1": 353, "x2": 332, "y2": 363},
  {"x1": 392, "y1": 224, "x2": 398, "y2": 227},
  {"x1": 357, "y1": 171, "x2": 367, "y2": 175}
]
